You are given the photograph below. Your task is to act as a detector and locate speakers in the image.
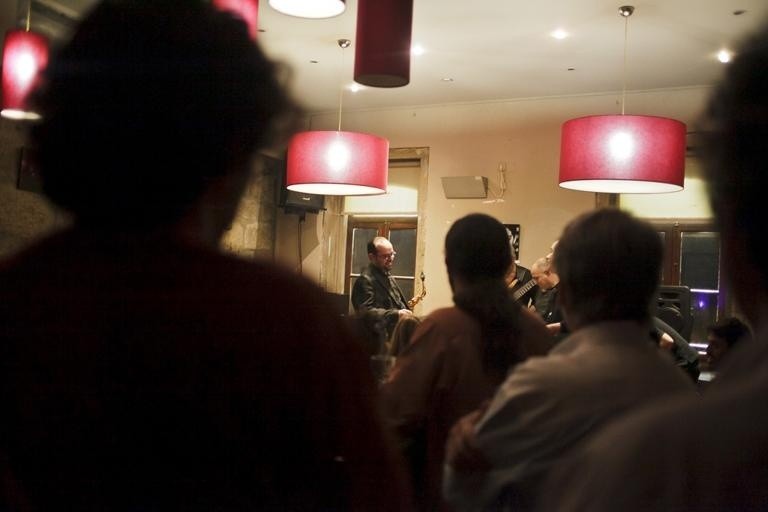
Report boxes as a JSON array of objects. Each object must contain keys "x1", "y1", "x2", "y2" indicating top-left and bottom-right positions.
[
  {"x1": 653, "y1": 286, "x2": 692, "y2": 343},
  {"x1": 277, "y1": 159, "x2": 325, "y2": 215}
]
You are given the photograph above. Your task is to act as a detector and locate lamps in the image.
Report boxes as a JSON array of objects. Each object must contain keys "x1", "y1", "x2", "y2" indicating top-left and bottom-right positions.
[
  {"x1": 0, "y1": 0, "x2": 47, "y2": 120},
  {"x1": 281, "y1": 34, "x2": 389, "y2": 200},
  {"x1": 551, "y1": 3, "x2": 690, "y2": 198},
  {"x1": 214, "y1": 0, "x2": 413, "y2": 88}
]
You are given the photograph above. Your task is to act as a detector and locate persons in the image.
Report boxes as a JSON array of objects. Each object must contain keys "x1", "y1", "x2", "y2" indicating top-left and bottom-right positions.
[
  {"x1": 375, "y1": 211, "x2": 558, "y2": 511},
  {"x1": 543, "y1": 239, "x2": 560, "y2": 336},
  {"x1": 1, "y1": 0, "x2": 423, "y2": 511},
  {"x1": 352, "y1": 232, "x2": 416, "y2": 344},
  {"x1": 529, "y1": 259, "x2": 557, "y2": 317},
  {"x1": 503, "y1": 254, "x2": 533, "y2": 307},
  {"x1": 705, "y1": 318, "x2": 752, "y2": 381},
  {"x1": 651, "y1": 317, "x2": 700, "y2": 386},
  {"x1": 530, "y1": 16, "x2": 765, "y2": 511},
  {"x1": 443, "y1": 206, "x2": 705, "y2": 510}
]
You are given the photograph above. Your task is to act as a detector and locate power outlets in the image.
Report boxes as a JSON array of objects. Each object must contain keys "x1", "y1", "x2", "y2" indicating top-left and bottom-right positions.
[{"x1": 497, "y1": 161, "x2": 505, "y2": 172}]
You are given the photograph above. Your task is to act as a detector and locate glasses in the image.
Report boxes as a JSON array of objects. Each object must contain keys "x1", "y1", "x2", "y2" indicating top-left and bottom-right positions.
[{"x1": 374, "y1": 250, "x2": 396, "y2": 260}]
